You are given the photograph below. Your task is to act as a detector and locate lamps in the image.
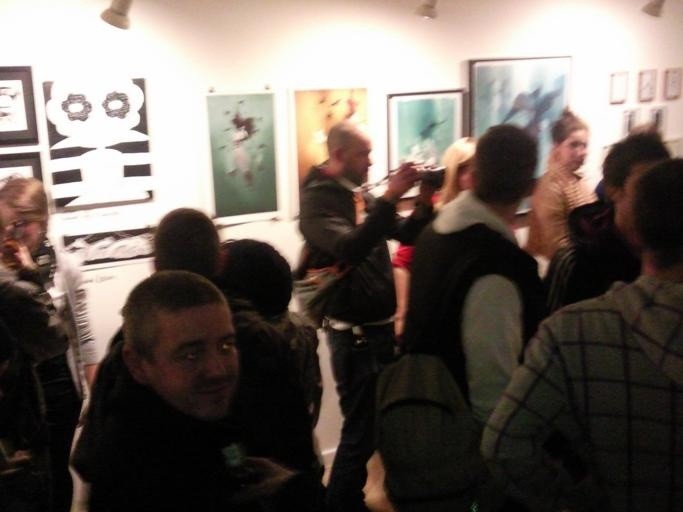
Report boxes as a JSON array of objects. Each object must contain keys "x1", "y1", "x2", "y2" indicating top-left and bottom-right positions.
[
  {"x1": 641, "y1": 1, "x2": 665, "y2": 18},
  {"x1": 415, "y1": 0, "x2": 438, "y2": 20},
  {"x1": 100, "y1": 0, "x2": 133, "y2": 29}
]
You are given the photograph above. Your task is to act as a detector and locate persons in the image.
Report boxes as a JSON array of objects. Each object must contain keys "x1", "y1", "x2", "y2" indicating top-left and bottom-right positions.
[
  {"x1": 394, "y1": 111, "x2": 683, "y2": 512},
  {"x1": 297, "y1": 123, "x2": 443, "y2": 512},
  {"x1": 0, "y1": 174, "x2": 100, "y2": 512},
  {"x1": 69, "y1": 209, "x2": 327, "y2": 512}
]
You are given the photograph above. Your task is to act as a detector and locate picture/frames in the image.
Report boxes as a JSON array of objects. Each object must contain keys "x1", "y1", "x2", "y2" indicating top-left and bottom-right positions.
[
  {"x1": 469, "y1": 56, "x2": 568, "y2": 217},
  {"x1": 609, "y1": 68, "x2": 682, "y2": 135},
  {"x1": 387, "y1": 86, "x2": 470, "y2": 177}
]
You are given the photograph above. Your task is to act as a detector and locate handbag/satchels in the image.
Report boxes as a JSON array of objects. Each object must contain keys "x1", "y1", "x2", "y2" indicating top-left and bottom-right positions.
[
  {"x1": 374, "y1": 356, "x2": 479, "y2": 511},
  {"x1": 289, "y1": 243, "x2": 353, "y2": 328}
]
